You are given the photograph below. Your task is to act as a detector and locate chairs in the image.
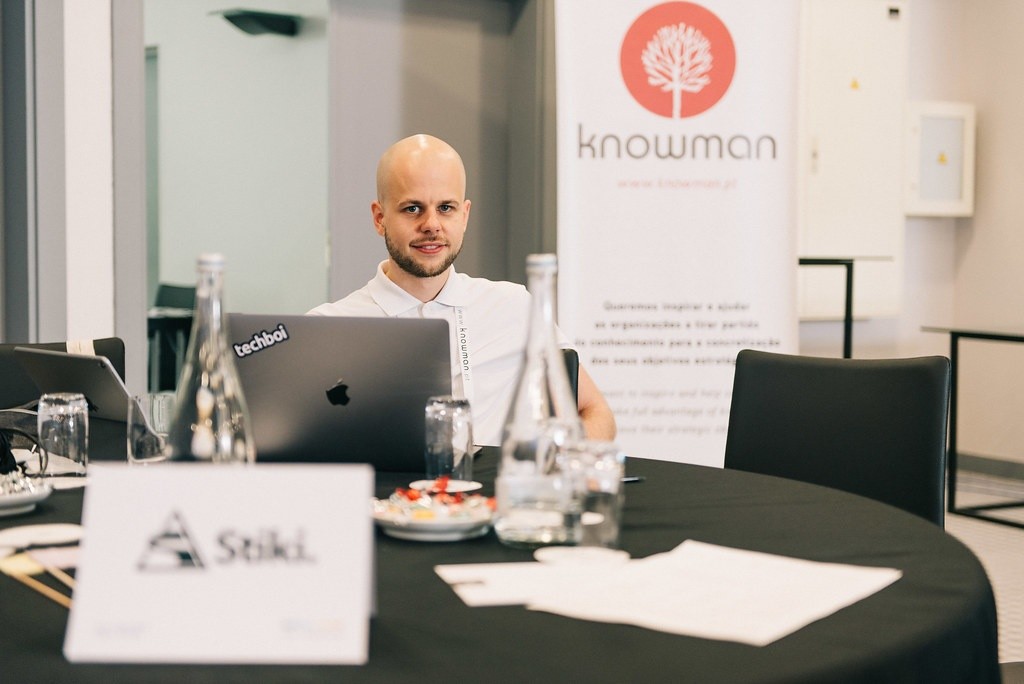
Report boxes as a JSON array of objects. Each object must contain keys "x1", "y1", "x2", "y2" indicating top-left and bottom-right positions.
[{"x1": 723, "y1": 347, "x2": 951, "y2": 531}]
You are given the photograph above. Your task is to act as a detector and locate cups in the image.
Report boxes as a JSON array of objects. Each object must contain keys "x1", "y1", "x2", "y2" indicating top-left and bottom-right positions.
[
  {"x1": 561, "y1": 438, "x2": 625, "y2": 547},
  {"x1": 424, "y1": 396, "x2": 475, "y2": 483},
  {"x1": 39, "y1": 392, "x2": 90, "y2": 476},
  {"x1": 126, "y1": 393, "x2": 177, "y2": 464}
]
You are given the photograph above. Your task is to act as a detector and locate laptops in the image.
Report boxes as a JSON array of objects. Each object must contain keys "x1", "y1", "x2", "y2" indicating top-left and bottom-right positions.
[
  {"x1": 223, "y1": 312, "x2": 482, "y2": 473},
  {"x1": 13, "y1": 346, "x2": 148, "y2": 426}
]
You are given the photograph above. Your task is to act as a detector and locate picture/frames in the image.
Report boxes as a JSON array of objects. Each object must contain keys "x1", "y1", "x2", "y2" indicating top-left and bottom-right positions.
[{"x1": 903, "y1": 100, "x2": 976, "y2": 217}]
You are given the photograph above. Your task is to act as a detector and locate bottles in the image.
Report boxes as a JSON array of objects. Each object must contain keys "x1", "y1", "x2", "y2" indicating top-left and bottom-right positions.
[
  {"x1": 167, "y1": 254, "x2": 256, "y2": 463},
  {"x1": 493, "y1": 252, "x2": 586, "y2": 545}
]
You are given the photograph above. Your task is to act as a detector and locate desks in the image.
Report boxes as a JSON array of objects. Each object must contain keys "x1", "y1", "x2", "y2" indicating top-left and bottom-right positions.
[{"x1": 0, "y1": 444, "x2": 1002, "y2": 684}]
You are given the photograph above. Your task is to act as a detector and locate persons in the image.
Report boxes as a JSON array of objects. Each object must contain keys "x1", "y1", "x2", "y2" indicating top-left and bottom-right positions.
[{"x1": 303, "y1": 135, "x2": 617, "y2": 452}]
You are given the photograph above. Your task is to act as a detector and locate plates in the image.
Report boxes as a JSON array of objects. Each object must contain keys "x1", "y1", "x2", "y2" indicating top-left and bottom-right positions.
[
  {"x1": 0, "y1": 487, "x2": 52, "y2": 516},
  {"x1": 533, "y1": 546, "x2": 630, "y2": 564},
  {"x1": 409, "y1": 479, "x2": 482, "y2": 492},
  {"x1": 374, "y1": 508, "x2": 493, "y2": 540}
]
